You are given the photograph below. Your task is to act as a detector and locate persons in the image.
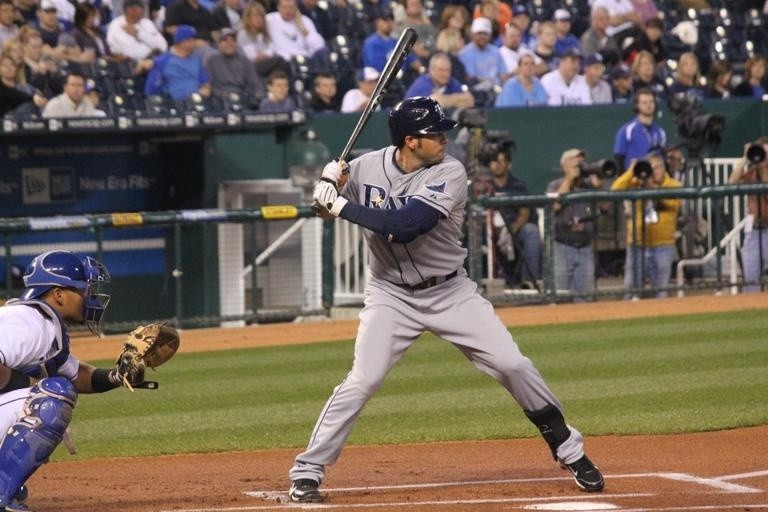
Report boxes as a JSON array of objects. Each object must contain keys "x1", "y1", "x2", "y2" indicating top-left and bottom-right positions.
[
  {"x1": 289, "y1": 95, "x2": 605, "y2": 503},
  {"x1": 0, "y1": 0, "x2": 767, "y2": 301},
  {"x1": 0, "y1": 248, "x2": 145, "y2": 511}
]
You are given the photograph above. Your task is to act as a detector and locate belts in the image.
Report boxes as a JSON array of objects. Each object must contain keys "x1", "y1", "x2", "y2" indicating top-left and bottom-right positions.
[{"x1": 395, "y1": 266, "x2": 458, "y2": 289}]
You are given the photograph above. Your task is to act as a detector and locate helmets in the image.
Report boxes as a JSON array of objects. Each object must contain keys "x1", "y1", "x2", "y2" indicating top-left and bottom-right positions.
[
  {"x1": 389, "y1": 95, "x2": 458, "y2": 144},
  {"x1": 22, "y1": 248, "x2": 112, "y2": 340}
]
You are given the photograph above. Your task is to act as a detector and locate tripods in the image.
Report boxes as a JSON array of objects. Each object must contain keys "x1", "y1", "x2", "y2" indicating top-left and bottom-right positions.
[{"x1": 663, "y1": 141, "x2": 745, "y2": 287}]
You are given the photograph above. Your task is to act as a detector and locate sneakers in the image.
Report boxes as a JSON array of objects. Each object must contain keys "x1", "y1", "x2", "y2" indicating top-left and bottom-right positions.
[
  {"x1": 289, "y1": 479, "x2": 325, "y2": 503},
  {"x1": 562, "y1": 455, "x2": 604, "y2": 493}
]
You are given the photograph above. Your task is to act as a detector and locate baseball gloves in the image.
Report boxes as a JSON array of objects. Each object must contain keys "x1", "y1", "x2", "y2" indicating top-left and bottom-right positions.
[{"x1": 115, "y1": 322, "x2": 179, "y2": 389}]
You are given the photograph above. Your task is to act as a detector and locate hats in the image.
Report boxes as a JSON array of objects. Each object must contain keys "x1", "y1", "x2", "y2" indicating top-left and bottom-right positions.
[{"x1": 39, "y1": 2, "x2": 629, "y2": 81}]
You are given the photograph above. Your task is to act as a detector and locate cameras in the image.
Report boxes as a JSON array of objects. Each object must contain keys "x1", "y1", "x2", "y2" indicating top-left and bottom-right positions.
[
  {"x1": 746, "y1": 143, "x2": 767, "y2": 164},
  {"x1": 576, "y1": 157, "x2": 619, "y2": 189},
  {"x1": 633, "y1": 161, "x2": 653, "y2": 180},
  {"x1": 461, "y1": 162, "x2": 544, "y2": 295}
]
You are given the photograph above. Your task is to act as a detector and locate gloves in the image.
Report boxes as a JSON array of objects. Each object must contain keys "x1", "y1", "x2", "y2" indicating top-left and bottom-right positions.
[
  {"x1": 322, "y1": 158, "x2": 352, "y2": 192},
  {"x1": 312, "y1": 180, "x2": 342, "y2": 210}
]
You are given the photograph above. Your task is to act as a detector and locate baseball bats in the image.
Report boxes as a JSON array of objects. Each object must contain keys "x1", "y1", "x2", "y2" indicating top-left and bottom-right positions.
[{"x1": 311, "y1": 27, "x2": 419, "y2": 213}]
